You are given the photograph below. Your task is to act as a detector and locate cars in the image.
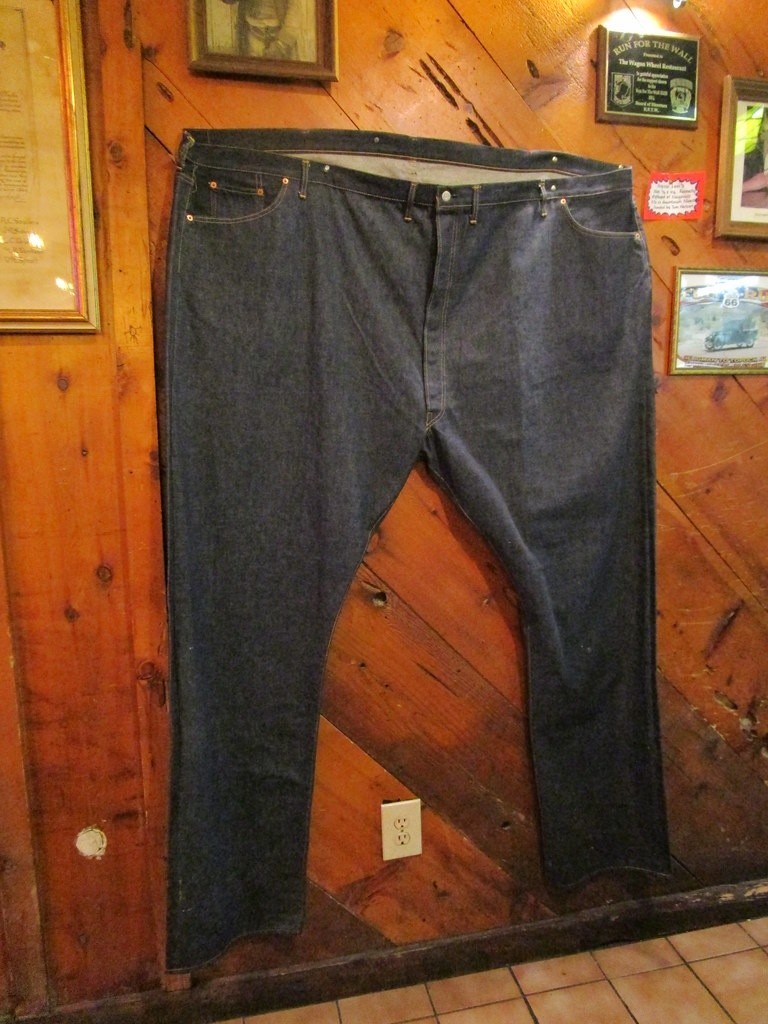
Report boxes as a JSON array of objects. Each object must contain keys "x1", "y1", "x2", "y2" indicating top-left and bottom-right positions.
[{"x1": 705, "y1": 318, "x2": 759, "y2": 352}]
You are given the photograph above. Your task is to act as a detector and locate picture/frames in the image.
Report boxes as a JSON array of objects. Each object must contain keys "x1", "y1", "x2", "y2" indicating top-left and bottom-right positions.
[
  {"x1": 667, "y1": 267, "x2": 768, "y2": 374},
  {"x1": 595, "y1": 25, "x2": 701, "y2": 130},
  {"x1": 715, "y1": 75, "x2": 768, "y2": 240},
  {"x1": 189, "y1": 0, "x2": 340, "y2": 81}
]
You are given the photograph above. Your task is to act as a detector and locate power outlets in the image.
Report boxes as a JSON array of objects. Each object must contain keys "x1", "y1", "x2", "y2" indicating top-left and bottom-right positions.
[{"x1": 380, "y1": 797, "x2": 422, "y2": 861}]
